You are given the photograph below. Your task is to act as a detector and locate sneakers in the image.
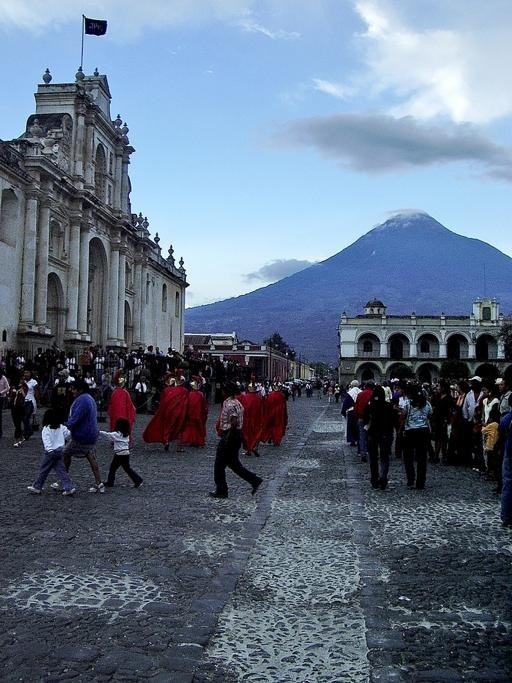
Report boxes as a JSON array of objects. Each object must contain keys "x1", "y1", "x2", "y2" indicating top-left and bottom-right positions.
[
  {"x1": 27, "y1": 486, "x2": 40, "y2": 494},
  {"x1": 63, "y1": 489, "x2": 75, "y2": 496}
]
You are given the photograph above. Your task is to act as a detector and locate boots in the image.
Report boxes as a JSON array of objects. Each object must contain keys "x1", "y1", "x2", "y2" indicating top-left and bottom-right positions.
[
  {"x1": 165, "y1": 435, "x2": 169, "y2": 450},
  {"x1": 252, "y1": 441, "x2": 259, "y2": 456},
  {"x1": 246, "y1": 442, "x2": 252, "y2": 455},
  {"x1": 176, "y1": 443, "x2": 185, "y2": 452}
]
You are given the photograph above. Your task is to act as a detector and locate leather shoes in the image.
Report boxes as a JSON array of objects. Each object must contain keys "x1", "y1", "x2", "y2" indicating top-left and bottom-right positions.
[
  {"x1": 251, "y1": 478, "x2": 263, "y2": 494},
  {"x1": 104, "y1": 482, "x2": 113, "y2": 487},
  {"x1": 407, "y1": 480, "x2": 413, "y2": 485},
  {"x1": 209, "y1": 490, "x2": 228, "y2": 498},
  {"x1": 373, "y1": 482, "x2": 379, "y2": 488},
  {"x1": 135, "y1": 478, "x2": 143, "y2": 487},
  {"x1": 418, "y1": 484, "x2": 425, "y2": 490},
  {"x1": 381, "y1": 482, "x2": 386, "y2": 489}
]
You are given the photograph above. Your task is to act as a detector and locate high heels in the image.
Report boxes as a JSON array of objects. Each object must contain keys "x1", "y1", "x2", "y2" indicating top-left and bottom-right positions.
[
  {"x1": 14, "y1": 441, "x2": 21, "y2": 448},
  {"x1": 51, "y1": 482, "x2": 62, "y2": 489},
  {"x1": 21, "y1": 439, "x2": 25, "y2": 445},
  {"x1": 89, "y1": 483, "x2": 104, "y2": 493}
]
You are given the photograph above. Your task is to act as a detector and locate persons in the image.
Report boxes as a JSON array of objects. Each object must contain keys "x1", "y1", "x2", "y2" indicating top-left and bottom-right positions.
[
  {"x1": 8, "y1": 377, "x2": 28, "y2": 447},
  {"x1": 478, "y1": 379, "x2": 501, "y2": 480},
  {"x1": 50, "y1": 380, "x2": 104, "y2": 494},
  {"x1": 397, "y1": 384, "x2": 433, "y2": 490},
  {"x1": 27, "y1": 411, "x2": 75, "y2": 496},
  {"x1": 235, "y1": 382, "x2": 261, "y2": 458},
  {"x1": 379, "y1": 375, "x2": 436, "y2": 406},
  {"x1": 109, "y1": 372, "x2": 135, "y2": 447},
  {"x1": 20, "y1": 371, "x2": 37, "y2": 441},
  {"x1": 61, "y1": 344, "x2": 267, "y2": 385},
  {"x1": 141, "y1": 378, "x2": 186, "y2": 450},
  {"x1": 30, "y1": 342, "x2": 66, "y2": 400},
  {"x1": 354, "y1": 380, "x2": 374, "y2": 460},
  {"x1": 178, "y1": 380, "x2": 206, "y2": 451},
  {"x1": 432, "y1": 379, "x2": 455, "y2": 464},
  {"x1": 499, "y1": 395, "x2": 511, "y2": 526},
  {"x1": 482, "y1": 410, "x2": 499, "y2": 475},
  {"x1": 0, "y1": 345, "x2": 34, "y2": 376},
  {"x1": 99, "y1": 420, "x2": 143, "y2": 488},
  {"x1": 209, "y1": 382, "x2": 262, "y2": 500},
  {"x1": 341, "y1": 381, "x2": 360, "y2": 448},
  {"x1": 263, "y1": 382, "x2": 287, "y2": 447},
  {"x1": 0, "y1": 371, "x2": 10, "y2": 432},
  {"x1": 266, "y1": 375, "x2": 343, "y2": 403},
  {"x1": 361, "y1": 386, "x2": 396, "y2": 488},
  {"x1": 447, "y1": 380, "x2": 476, "y2": 466}
]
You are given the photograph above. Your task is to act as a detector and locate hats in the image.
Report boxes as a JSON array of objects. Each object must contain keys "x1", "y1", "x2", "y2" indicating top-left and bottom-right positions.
[
  {"x1": 495, "y1": 378, "x2": 503, "y2": 384},
  {"x1": 468, "y1": 376, "x2": 482, "y2": 382},
  {"x1": 222, "y1": 379, "x2": 236, "y2": 395}
]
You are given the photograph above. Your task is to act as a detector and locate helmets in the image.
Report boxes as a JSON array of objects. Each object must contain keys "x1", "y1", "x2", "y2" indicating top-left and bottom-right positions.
[
  {"x1": 190, "y1": 380, "x2": 201, "y2": 390},
  {"x1": 176, "y1": 376, "x2": 184, "y2": 385},
  {"x1": 248, "y1": 383, "x2": 257, "y2": 392},
  {"x1": 272, "y1": 383, "x2": 283, "y2": 391},
  {"x1": 117, "y1": 377, "x2": 125, "y2": 387},
  {"x1": 165, "y1": 378, "x2": 175, "y2": 386},
  {"x1": 235, "y1": 382, "x2": 242, "y2": 389}
]
